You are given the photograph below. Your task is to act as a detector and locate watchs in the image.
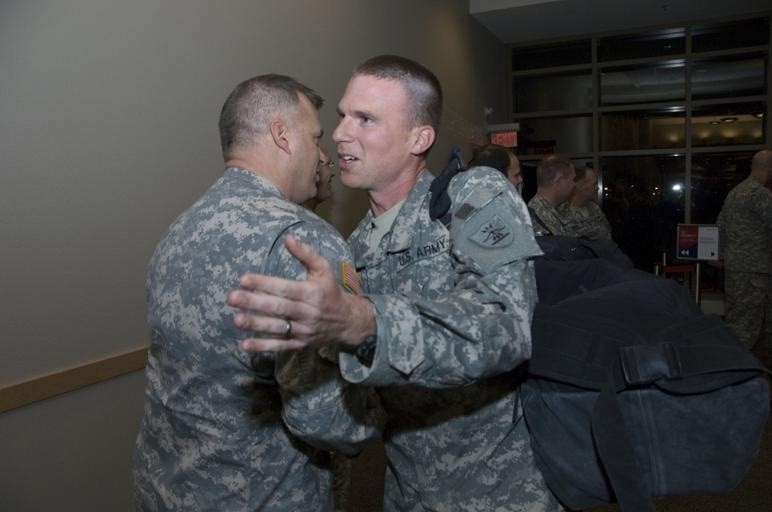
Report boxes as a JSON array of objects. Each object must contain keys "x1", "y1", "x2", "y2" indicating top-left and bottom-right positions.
[{"x1": 356, "y1": 332, "x2": 378, "y2": 366}]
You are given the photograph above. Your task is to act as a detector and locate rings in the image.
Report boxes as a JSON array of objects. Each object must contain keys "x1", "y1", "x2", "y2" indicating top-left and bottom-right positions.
[{"x1": 282, "y1": 316, "x2": 292, "y2": 338}]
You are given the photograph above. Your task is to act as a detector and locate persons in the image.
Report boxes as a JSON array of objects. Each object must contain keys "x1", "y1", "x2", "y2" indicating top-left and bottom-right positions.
[
  {"x1": 297, "y1": 138, "x2": 355, "y2": 511},
  {"x1": 718, "y1": 148, "x2": 772, "y2": 355},
  {"x1": 526, "y1": 152, "x2": 578, "y2": 239},
  {"x1": 226, "y1": 52, "x2": 564, "y2": 511},
  {"x1": 469, "y1": 143, "x2": 523, "y2": 193},
  {"x1": 556, "y1": 163, "x2": 613, "y2": 240},
  {"x1": 133, "y1": 71, "x2": 388, "y2": 512}
]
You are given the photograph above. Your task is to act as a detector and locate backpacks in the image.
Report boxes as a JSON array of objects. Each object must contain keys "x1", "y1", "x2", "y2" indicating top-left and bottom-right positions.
[{"x1": 429, "y1": 163, "x2": 772, "y2": 511}]
